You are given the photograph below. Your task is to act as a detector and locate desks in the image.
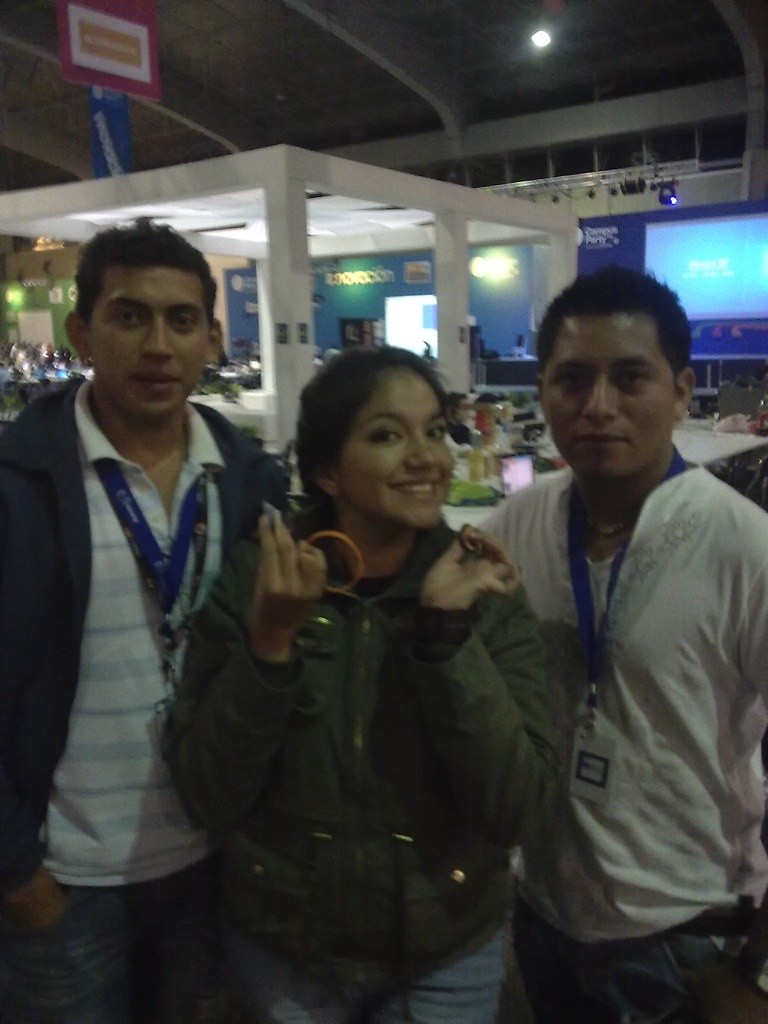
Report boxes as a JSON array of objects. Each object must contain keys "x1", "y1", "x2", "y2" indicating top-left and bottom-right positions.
[{"x1": 673, "y1": 431, "x2": 768, "y2": 497}]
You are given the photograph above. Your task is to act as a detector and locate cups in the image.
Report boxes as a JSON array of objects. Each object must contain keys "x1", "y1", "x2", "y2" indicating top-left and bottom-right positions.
[{"x1": 468, "y1": 448, "x2": 496, "y2": 482}]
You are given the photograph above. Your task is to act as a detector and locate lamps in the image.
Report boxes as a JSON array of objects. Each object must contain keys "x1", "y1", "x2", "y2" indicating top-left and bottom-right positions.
[
  {"x1": 658, "y1": 185, "x2": 677, "y2": 205},
  {"x1": 620, "y1": 178, "x2": 646, "y2": 195}
]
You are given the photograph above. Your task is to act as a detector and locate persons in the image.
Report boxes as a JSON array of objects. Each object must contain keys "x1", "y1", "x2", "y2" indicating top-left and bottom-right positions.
[
  {"x1": 162, "y1": 345, "x2": 549, "y2": 1024},
  {"x1": 473, "y1": 263, "x2": 768, "y2": 1024},
  {"x1": 0, "y1": 219, "x2": 289, "y2": 1024},
  {"x1": 323, "y1": 343, "x2": 348, "y2": 364},
  {"x1": 512, "y1": 334, "x2": 527, "y2": 359}
]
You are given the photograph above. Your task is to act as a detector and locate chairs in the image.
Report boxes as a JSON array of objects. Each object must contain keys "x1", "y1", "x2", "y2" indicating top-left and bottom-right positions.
[{"x1": 717, "y1": 383, "x2": 764, "y2": 421}]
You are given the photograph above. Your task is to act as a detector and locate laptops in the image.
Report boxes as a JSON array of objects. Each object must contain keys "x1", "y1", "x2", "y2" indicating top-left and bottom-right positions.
[{"x1": 499, "y1": 452, "x2": 536, "y2": 501}]
